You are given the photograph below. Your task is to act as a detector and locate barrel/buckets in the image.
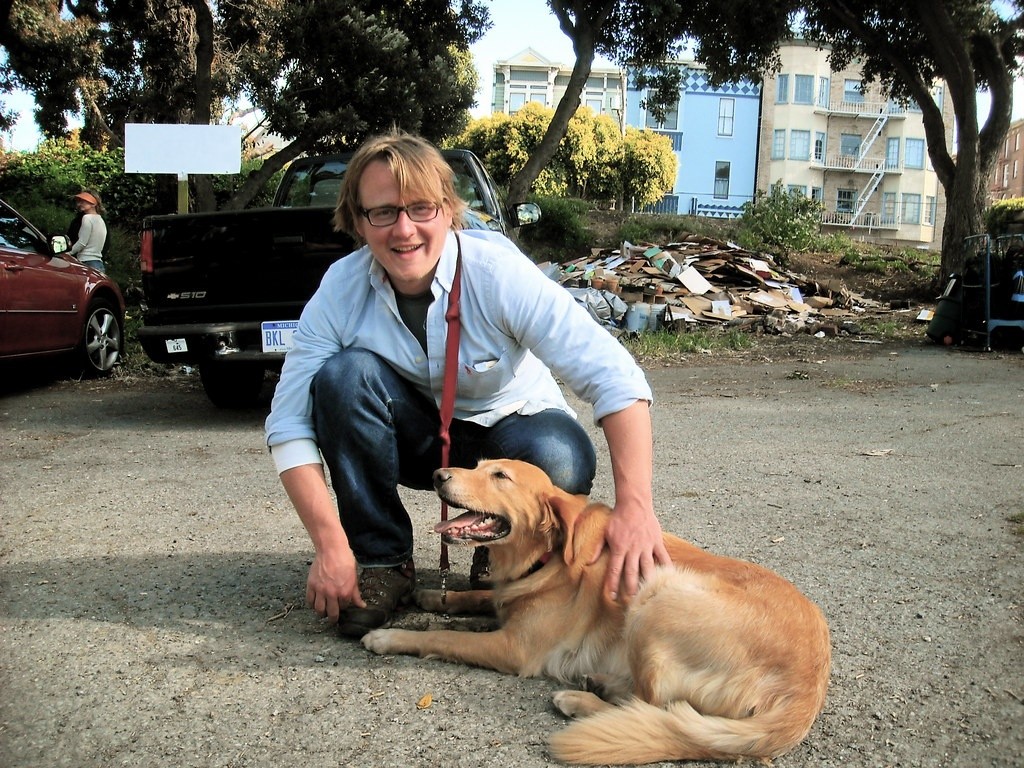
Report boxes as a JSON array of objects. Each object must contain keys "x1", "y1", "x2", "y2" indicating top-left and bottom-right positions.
[
  {"x1": 626, "y1": 302, "x2": 650, "y2": 331},
  {"x1": 648, "y1": 304, "x2": 667, "y2": 330}
]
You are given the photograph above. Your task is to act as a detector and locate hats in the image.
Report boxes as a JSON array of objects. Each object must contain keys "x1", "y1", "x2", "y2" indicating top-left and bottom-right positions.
[{"x1": 75, "y1": 192, "x2": 98, "y2": 207}]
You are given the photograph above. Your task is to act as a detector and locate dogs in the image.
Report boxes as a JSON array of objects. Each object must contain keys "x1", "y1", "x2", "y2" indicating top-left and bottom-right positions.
[{"x1": 359, "y1": 459, "x2": 832, "y2": 768}]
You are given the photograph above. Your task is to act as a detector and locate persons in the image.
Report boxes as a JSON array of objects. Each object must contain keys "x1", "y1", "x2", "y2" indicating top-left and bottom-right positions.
[
  {"x1": 265, "y1": 125, "x2": 675, "y2": 638},
  {"x1": 54, "y1": 240, "x2": 62, "y2": 253},
  {"x1": 65, "y1": 190, "x2": 107, "y2": 274}
]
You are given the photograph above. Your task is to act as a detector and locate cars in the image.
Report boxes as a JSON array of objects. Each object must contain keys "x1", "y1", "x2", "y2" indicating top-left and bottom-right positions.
[{"x1": 0, "y1": 195, "x2": 126, "y2": 395}]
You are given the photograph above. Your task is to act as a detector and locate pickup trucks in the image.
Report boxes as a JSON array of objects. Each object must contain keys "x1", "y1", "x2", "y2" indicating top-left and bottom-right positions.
[{"x1": 135, "y1": 146, "x2": 543, "y2": 413}]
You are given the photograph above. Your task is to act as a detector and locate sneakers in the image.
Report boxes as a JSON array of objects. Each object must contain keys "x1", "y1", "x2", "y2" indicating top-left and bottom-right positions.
[
  {"x1": 338, "y1": 555, "x2": 417, "y2": 640},
  {"x1": 470, "y1": 562, "x2": 497, "y2": 590}
]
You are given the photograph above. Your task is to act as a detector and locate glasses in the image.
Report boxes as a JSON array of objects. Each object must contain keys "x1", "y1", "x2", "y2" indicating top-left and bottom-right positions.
[{"x1": 358, "y1": 196, "x2": 446, "y2": 227}]
